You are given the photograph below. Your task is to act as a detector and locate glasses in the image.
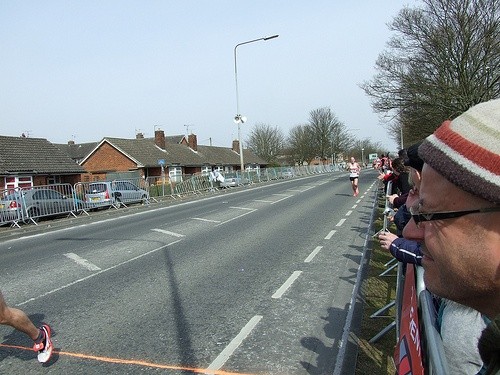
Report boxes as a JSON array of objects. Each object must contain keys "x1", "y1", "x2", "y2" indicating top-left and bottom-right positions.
[{"x1": 409, "y1": 200, "x2": 499, "y2": 229}]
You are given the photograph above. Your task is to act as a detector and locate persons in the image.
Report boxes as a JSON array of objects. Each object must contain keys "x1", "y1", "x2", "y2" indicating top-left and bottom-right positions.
[
  {"x1": 402, "y1": 97, "x2": 500, "y2": 375},
  {"x1": 372, "y1": 140, "x2": 494, "y2": 375},
  {"x1": 0, "y1": 289, "x2": 55, "y2": 364},
  {"x1": 346, "y1": 156, "x2": 361, "y2": 197}
]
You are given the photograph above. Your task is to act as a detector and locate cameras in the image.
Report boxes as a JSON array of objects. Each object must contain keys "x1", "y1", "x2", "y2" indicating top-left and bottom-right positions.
[{"x1": 384, "y1": 207, "x2": 396, "y2": 217}]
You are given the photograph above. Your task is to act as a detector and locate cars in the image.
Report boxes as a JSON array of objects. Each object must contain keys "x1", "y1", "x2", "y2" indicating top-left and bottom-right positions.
[
  {"x1": 0, "y1": 189, "x2": 86, "y2": 225},
  {"x1": 220, "y1": 173, "x2": 251, "y2": 188},
  {"x1": 279, "y1": 168, "x2": 295, "y2": 178},
  {"x1": 84, "y1": 181, "x2": 150, "y2": 210}
]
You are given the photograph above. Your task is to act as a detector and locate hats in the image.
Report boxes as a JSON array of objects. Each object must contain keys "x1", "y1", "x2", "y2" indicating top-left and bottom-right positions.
[
  {"x1": 419, "y1": 98, "x2": 499, "y2": 202},
  {"x1": 404, "y1": 142, "x2": 424, "y2": 172}
]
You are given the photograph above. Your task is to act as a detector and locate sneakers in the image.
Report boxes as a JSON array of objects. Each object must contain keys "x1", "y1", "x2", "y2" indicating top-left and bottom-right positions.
[{"x1": 32, "y1": 325, "x2": 53, "y2": 364}]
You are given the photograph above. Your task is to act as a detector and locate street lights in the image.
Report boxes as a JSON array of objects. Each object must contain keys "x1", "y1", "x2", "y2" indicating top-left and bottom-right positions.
[
  {"x1": 361, "y1": 148, "x2": 364, "y2": 162},
  {"x1": 234, "y1": 34, "x2": 279, "y2": 170}
]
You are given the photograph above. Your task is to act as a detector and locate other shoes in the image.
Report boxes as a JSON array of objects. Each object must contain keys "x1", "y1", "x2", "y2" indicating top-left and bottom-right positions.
[{"x1": 353, "y1": 191, "x2": 359, "y2": 197}]
